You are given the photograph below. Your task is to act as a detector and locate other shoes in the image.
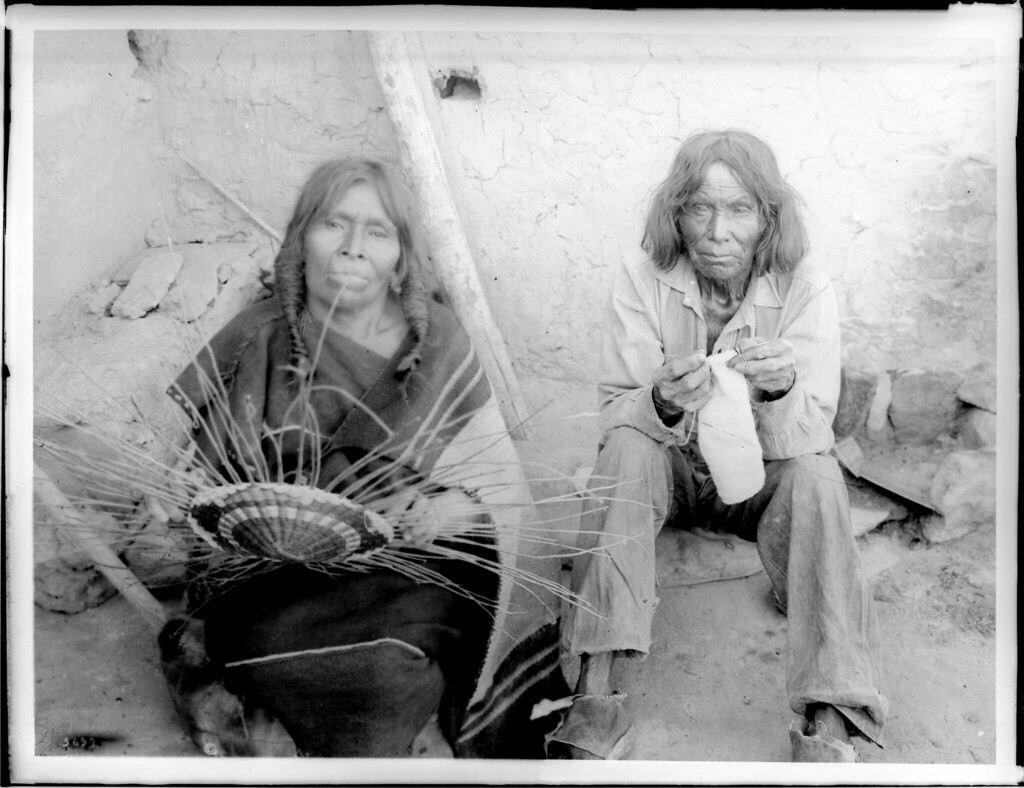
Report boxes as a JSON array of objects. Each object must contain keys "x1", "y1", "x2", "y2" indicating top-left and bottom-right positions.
[
  {"x1": 529, "y1": 687, "x2": 638, "y2": 762},
  {"x1": 786, "y1": 716, "x2": 865, "y2": 763}
]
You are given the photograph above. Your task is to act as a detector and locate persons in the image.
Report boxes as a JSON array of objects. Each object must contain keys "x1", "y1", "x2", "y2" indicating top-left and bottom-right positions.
[
  {"x1": 552, "y1": 129, "x2": 891, "y2": 762},
  {"x1": 157, "y1": 156, "x2": 501, "y2": 759}
]
